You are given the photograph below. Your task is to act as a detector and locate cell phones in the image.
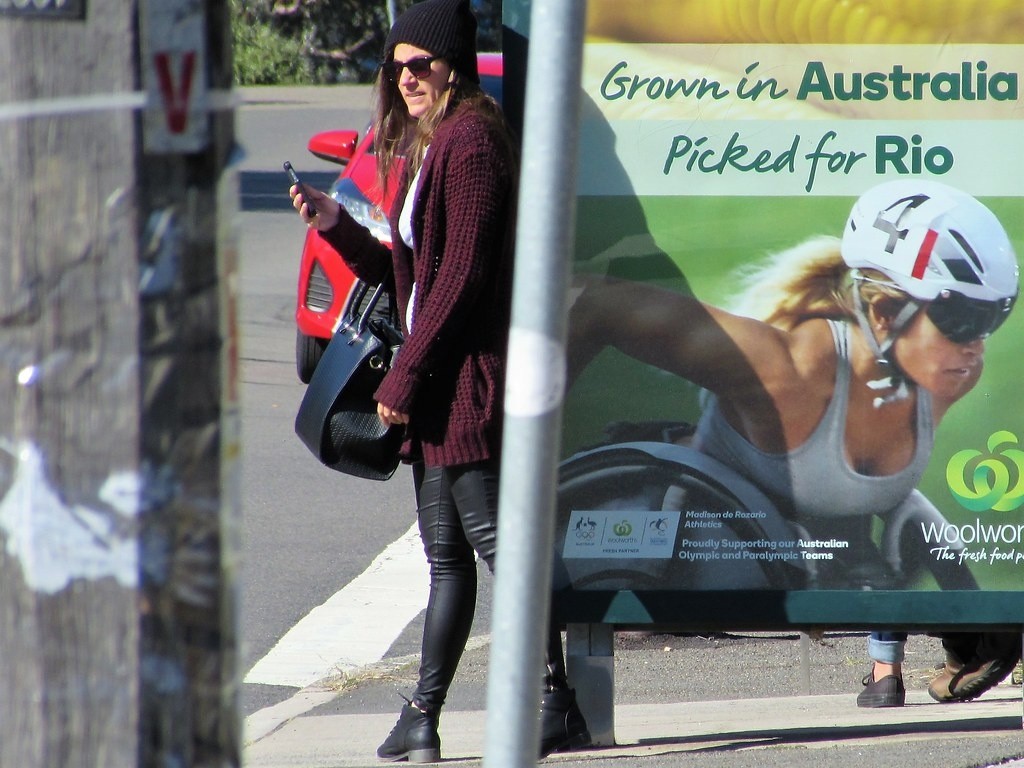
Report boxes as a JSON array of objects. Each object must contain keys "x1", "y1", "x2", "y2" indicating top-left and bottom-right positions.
[{"x1": 283, "y1": 161, "x2": 317, "y2": 219}]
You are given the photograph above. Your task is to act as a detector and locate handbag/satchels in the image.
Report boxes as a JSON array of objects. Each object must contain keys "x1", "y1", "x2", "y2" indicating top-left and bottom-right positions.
[{"x1": 294, "y1": 247, "x2": 410, "y2": 480}]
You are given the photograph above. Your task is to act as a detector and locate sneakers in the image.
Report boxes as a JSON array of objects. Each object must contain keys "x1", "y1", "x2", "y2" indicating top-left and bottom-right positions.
[{"x1": 929, "y1": 646, "x2": 1023, "y2": 702}]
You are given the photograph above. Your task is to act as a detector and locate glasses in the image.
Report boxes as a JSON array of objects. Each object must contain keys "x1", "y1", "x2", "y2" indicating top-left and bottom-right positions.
[
  {"x1": 380, "y1": 58, "x2": 437, "y2": 80},
  {"x1": 928, "y1": 287, "x2": 1013, "y2": 338}
]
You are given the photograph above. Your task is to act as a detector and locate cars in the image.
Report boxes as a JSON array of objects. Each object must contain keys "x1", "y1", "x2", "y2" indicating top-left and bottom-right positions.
[{"x1": 296, "y1": 52, "x2": 503, "y2": 385}]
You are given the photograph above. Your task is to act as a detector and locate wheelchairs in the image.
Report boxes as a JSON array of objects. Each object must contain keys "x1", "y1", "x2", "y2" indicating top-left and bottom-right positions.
[{"x1": 555, "y1": 420, "x2": 980, "y2": 589}]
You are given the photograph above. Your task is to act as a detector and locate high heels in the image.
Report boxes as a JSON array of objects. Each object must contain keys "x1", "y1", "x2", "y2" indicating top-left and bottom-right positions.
[
  {"x1": 539, "y1": 684, "x2": 592, "y2": 759},
  {"x1": 376, "y1": 689, "x2": 440, "y2": 763}
]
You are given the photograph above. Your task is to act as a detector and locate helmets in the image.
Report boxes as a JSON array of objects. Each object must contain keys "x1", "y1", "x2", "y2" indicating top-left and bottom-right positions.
[{"x1": 841, "y1": 176, "x2": 1022, "y2": 343}]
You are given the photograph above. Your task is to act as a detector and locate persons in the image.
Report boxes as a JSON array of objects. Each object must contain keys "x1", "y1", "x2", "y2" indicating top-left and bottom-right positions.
[
  {"x1": 569, "y1": 179, "x2": 1019, "y2": 585},
  {"x1": 289, "y1": 1, "x2": 593, "y2": 760},
  {"x1": 856, "y1": 632, "x2": 1024, "y2": 708}
]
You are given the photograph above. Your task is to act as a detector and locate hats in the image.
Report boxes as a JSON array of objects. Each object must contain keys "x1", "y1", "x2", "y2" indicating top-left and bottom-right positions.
[{"x1": 386, "y1": 1, "x2": 480, "y2": 84}]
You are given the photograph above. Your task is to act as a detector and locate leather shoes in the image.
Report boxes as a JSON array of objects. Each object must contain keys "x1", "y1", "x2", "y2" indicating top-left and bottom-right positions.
[{"x1": 854, "y1": 662, "x2": 906, "y2": 708}]
